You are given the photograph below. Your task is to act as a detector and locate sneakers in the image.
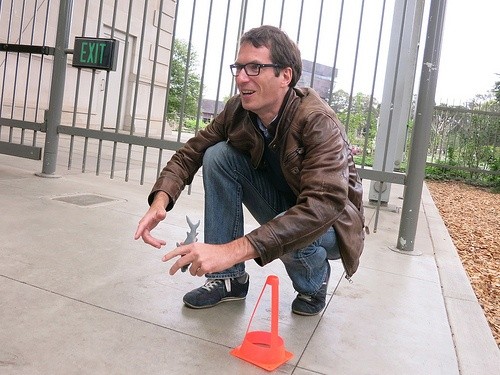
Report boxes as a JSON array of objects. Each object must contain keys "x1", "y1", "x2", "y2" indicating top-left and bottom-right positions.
[
  {"x1": 183, "y1": 270, "x2": 249, "y2": 309},
  {"x1": 292, "y1": 257, "x2": 331, "y2": 317}
]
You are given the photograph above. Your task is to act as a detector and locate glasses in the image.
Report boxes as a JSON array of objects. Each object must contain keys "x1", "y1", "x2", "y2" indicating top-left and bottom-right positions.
[{"x1": 230, "y1": 62, "x2": 288, "y2": 77}]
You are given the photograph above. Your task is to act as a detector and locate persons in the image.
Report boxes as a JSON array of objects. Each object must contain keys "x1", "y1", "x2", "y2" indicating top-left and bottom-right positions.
[{"x1": 134, "y1": 26, "x2": 365, "y2": 316}]
